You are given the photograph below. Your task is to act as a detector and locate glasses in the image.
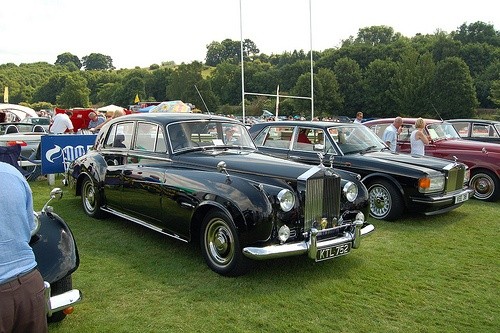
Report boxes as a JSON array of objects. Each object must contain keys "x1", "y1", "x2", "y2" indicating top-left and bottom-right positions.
[{"x1": 106, "y1": 116, "x2": 111, "y2": 118}]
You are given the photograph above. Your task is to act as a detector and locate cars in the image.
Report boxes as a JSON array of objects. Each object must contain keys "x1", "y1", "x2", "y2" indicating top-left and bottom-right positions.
[
  {"x1": 62, "y1": 113, "x2": 375, "y2": 276},
  {"x1": 346, "y1": 118, "x2": 500, "y2": 202},
  {"x1": 28, "y1": 187, "x2": 82, "y2": 324},
  {"x1": 228, "y1": 119, "x2": 476, "y2": 222},
  {"x1": 0, "y1": 99, "x2": 226, "y2": 166},
  {"x1": 435, "y1": 119, "x2": 500, "y2": 144}
]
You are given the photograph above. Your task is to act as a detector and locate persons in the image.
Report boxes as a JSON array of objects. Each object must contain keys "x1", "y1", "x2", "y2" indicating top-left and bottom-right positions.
[
  {"x1": 224, "y1": 128, "x2": 237, "y2": 144},
  {"x1": 0, "y1": 161, "x2": 48, "y2": 333},
  {"x1": 104, "y1": 132, "x2": 127, "y2": 165},
  {"x1": 382, "y1": 116, "x2": 403, "y2": 152},
  {"x1": 49, "y1": 110, "x2": 75, "y2": 134},
  {"x1": 266, "y1": 111, "x2": 339, "y2": 123},
  {"x1": 353, "y1": 111, "x2": 363, "y2": 123},
  {"x1": 411, "y1": 118, "x2": 432, "y2": 156},
  {"x1": 88, "y1": 107, "x2": 132, "y2": 134}
]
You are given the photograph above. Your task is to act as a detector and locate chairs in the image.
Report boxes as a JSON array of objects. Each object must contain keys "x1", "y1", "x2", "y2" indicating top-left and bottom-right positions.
[
  {"x1": 0, "y1": 144, "x2": 37, "y2": 180},
  {"x1": 291, "y1": 134, "x2": 312, "y2": 144}
]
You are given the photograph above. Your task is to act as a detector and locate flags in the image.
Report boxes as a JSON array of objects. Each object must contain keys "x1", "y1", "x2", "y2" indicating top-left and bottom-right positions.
[
  {"x1": 4, "y1": 86, "x2": 9, "y2": 101},
  {"x1": 135, "y1": 93, "x2": 140, "y2": 102}
]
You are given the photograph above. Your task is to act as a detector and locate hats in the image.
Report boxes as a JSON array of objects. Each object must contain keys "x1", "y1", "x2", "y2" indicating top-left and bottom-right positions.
[
  {"x1": 225, "y1": 127, "x2": 235, "y2": 133},
  {"x1": 88, "y1": 112, "x2": 97, "y2": 119}
]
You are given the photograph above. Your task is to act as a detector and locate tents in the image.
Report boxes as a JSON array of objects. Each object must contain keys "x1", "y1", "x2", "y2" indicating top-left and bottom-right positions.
[{"x1": 97, "y1": 104, "x2": 126, "y2": 119}]
